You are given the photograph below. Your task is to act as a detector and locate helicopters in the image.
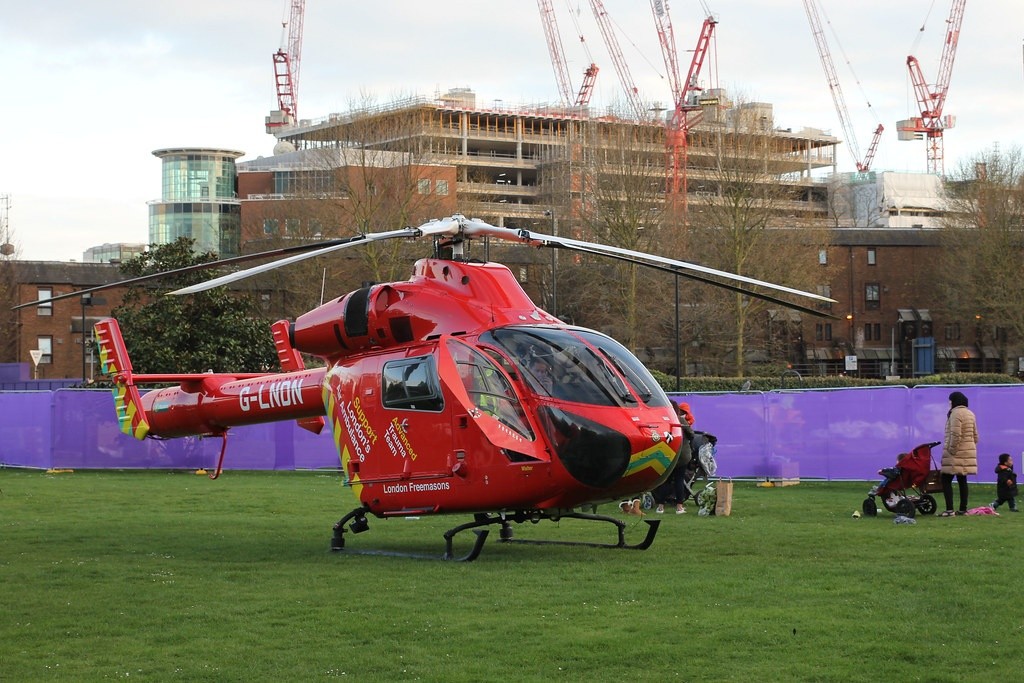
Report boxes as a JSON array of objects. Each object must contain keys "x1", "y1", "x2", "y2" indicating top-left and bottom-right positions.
[{"x1": 10, "y1": 212, "x2": 851, "y2": 565}]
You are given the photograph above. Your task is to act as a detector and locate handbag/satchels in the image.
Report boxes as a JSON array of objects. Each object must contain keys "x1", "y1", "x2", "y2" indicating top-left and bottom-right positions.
[
  {"x1": 698, "y1": 481, "x2": 717, "y2": 516},
  {"x1": 716, "y1": 474, "x2": 733, "y2": 516},
  {"x1": 919, "y1": 455, "x2": 944, "y2": 493}
]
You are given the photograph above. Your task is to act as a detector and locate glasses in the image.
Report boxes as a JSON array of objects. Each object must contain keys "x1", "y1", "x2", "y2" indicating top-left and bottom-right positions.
[{"x1": 532, "y1": 367, "x2": 545, "y2": 373}]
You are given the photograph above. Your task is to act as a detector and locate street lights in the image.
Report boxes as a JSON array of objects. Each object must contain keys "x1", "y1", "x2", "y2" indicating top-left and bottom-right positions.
[{"x1": 544, "y1": 211, "x2": 557, "y2": 317}]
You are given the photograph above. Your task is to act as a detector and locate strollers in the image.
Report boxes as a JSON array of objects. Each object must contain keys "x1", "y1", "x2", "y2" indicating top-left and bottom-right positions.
[
  {"x1": 642, "y1": 430, "x2": 717, "y2": 510},
  {"x1": 862, "y1": 441, "x2": 941, "y2": 518}
]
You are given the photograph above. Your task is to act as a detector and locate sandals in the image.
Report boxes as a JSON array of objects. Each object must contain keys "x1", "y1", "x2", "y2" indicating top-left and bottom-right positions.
[
  {"x1": 955, "y1": 511, "x2": 968, "y2": 516},
  {"x1": 936, "y1": 510, "x2": 955, "y2": 517}
]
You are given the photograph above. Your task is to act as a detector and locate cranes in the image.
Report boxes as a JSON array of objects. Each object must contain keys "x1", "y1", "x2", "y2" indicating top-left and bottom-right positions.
[
  {"x1": 536, "y1": 0, "x2": 600, "y2": 121},
  {"x1": 265, "y1": 0, "x2": 304, "y2": 133},
  {"x1": 650, "y1": 0, "x2": 717, "y2": 224},
  {"x1": 590, "y1": 0, "x2": 649, "y2": 124},
  {"x1": 897, "y1": 0, "x2": 969, "y2": 179},
  {"x1": 804, "y1": 0, "x2": 885, "y2": 172}
]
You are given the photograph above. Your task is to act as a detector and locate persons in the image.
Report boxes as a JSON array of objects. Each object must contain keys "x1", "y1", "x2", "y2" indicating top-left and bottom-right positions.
[
  {"x1": 617, "y1": 401, "x2": 696, "y2": 516},
  {"x1": 990, "y1": 453, "x2": 1019, "y2": 511},
  {"x1": 868, "y1": 453, "x2": 906, "y2": 492},
  {"x1": 939, "y1": 390, "x2": 979, "y2": 517},
  {"x1": 505, "y1": 359, "x2": 554, "y2": 440}
]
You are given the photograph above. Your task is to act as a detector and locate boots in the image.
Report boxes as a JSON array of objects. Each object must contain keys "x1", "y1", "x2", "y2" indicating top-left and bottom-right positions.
[
  {"x1": 619, "y1": 501, "x2": 628, "y2": 512},
  {"x1": 627, "y1": 498, "x2": 645, "y2": 515}
]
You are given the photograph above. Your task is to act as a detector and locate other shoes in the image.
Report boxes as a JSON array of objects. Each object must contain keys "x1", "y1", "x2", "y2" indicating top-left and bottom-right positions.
[
  {"x1": 1010, "y1": 508, "x2": 1019, "y2": 512},
  {"x1": 676, "y1": 508, "x2": 687, "y2": 514},
  {"x1": 656, "y1": 507, "x2": 664, "y2": 514},
  {"x1": 873, "y1": 486, "x2": 878, "y2": 491},
  {"x1": 988, "y1": 503, "x2": 996, "y2": 511}
]
